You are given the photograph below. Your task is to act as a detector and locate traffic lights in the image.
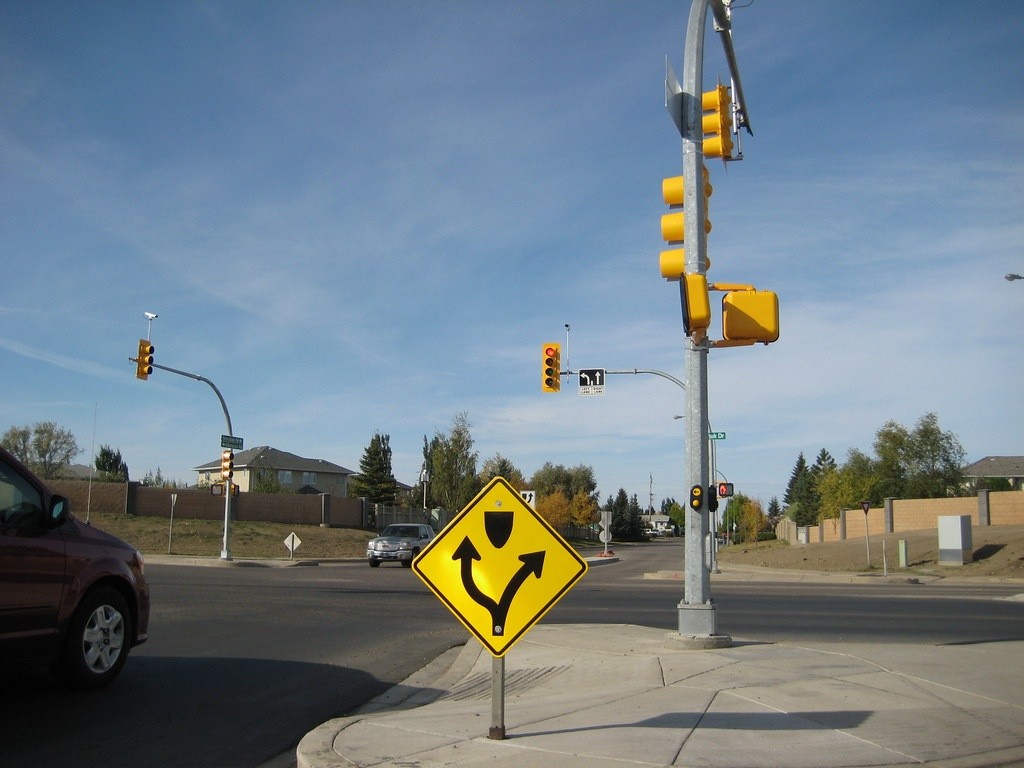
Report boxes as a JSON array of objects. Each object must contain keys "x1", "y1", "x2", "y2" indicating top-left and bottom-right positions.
[
  {"x1": 655, "y1": 162, "x2": 715, "y2": 281},
  {"x1": 221, "y1": 449, "x2": 235, "y2": 481},
  {"x1": 702, "y1": 80, "x2": 733, "y2": 171},
  {"x1": 135, "y1": 338, "x2": 153, "y2": 380},
  {"x1": 543, "y1": 339, "x2": 563, "y2": 392},
  {"x1": 720, "y1": 483, "x2": 733, "y2": 499}
]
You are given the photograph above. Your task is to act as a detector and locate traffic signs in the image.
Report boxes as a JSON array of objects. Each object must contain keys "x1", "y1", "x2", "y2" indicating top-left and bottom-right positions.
[
  {"x1": 220, "y1": 434, "x2": 244, "y2": 451},
  {"x1": 579, "y1": 368, "x2": 606, "y2": 395}
]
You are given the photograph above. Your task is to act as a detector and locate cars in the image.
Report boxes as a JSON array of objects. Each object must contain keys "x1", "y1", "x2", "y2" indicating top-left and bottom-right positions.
[{"x1": 1, "y1": 446, "x2": 151, "y2": 686}]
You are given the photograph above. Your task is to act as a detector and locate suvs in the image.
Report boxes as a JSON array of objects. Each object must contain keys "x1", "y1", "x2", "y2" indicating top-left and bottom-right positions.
[{"x1": 365, "y1": 520, "x2": 431, "y2": 568}]
